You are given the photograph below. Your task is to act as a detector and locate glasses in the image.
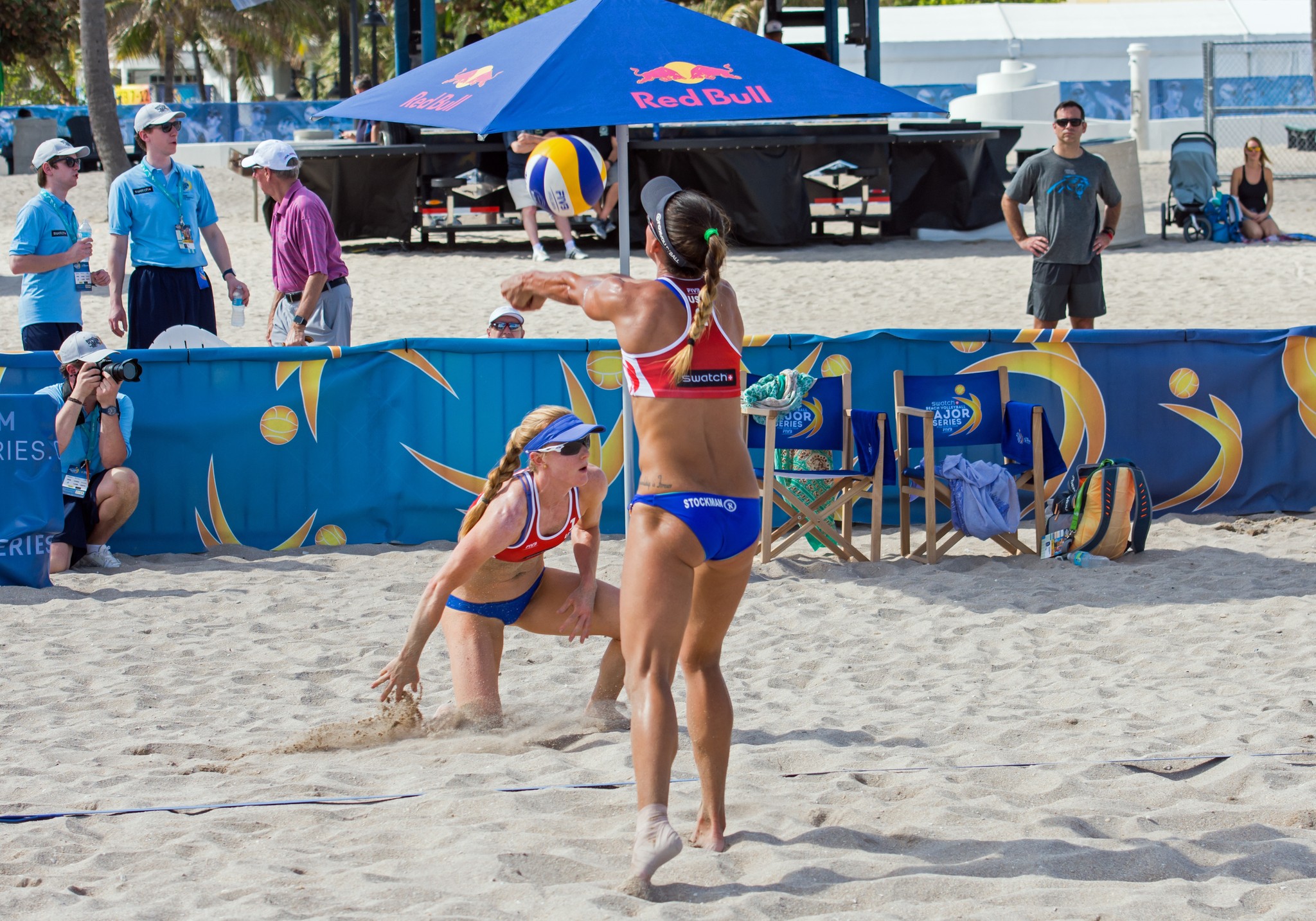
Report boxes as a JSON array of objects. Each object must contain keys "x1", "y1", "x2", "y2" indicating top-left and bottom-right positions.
[
  {"x1": 526, "y1": 434, "x2": 590, "y2": 456},
  {"x1": 252, "y1": 165, "x2": 263, "y2": 175},
  {"x1": 1247, "y1": 147, "x2": 1261, "y2": 152},
  {"x1": 136, "y1": 121, "x2": 182, "y2": 133},
  {"x1": 1055, "y1": 118, "x2": 1085, "y2": 128},
  {"x1": 491, "y1": 321, "x2": 522, "y2": 331},
  {"x1": 49, "y1": 157, "x2": 81, "y2": 169}
]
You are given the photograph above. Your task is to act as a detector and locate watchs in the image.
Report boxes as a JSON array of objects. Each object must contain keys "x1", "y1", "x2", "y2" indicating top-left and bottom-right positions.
[
  {"x1": 222, "y1": 268, "x2": 236, "y2": 281},
  {"x1": 293, "y1": 315, "x2": 308, "y2": 326},
  {"x1": 100, "y1": 405, "x2": 117, "y2": 416}
]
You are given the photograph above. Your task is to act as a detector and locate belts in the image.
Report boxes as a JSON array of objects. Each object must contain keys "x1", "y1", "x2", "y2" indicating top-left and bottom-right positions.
[{"x1": 285, "y1": 276, "x2": 346, "y2": 303}]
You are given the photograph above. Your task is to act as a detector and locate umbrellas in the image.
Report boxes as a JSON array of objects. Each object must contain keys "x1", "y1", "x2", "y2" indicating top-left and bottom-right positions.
[{"x1": 315, "y1": 0, "x2": 947, "y2": 536}]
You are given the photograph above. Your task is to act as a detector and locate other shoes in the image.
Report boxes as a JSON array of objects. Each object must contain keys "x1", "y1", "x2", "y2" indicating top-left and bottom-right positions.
[
  {"x1": 597, "y1": 217, "x2": 615, "y2": 233},
  {"x1": 566, "y1": 246, "x2": 588, "y2": 260},
  {"x1": 532, "y1": 246, "x2": 549, "y2": 261},
  {"x1": 591, "y1": 217, "x2": 607, "y2": 239}
]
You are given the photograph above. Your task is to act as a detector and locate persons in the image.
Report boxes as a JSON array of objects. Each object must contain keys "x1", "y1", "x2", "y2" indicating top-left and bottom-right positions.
[
  {"x1": 34, "y1": 331, "x2": 139, "y2": 573},
  {"x1": 476, "y1": 306, "x2": 525, "y2": 339},
  {"x1": 9, "y1": 137, "x2": 111, "y2": 352},
  {"x1": 107, "y1": 103, "x2": 250, "y2": 349},
  {"x1": 1000, "y1": 100, "x2": 1122, "y2": 329},
  {"x1": 241, "y1": 138, "x2": 353, "y2": 346},
  {"x1": 342, "y1": 74, "x2": 381, "y2": 143},
  {"x1": 498, "y1": 177, "x2": 764, "y2": 898},
  {"x1": 503, "y1": 126, "x2": 618, "y2": 261},
  {"x1": 1230, "y1": 137, "x2": 1281, "y2": 241}
]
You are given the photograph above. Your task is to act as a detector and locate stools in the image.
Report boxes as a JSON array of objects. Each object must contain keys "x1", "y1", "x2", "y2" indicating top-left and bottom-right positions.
[
  {"x1": 431, "y1": 177, "x2": 467, "y2": 227},
  {"x1": 846, "y1": 166, "x2": 878, "y2": 216}
]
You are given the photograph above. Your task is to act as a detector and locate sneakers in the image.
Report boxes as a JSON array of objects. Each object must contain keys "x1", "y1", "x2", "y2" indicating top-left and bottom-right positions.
[{"x1": 80, "y1": 544, "x2": 121, "y2": 568}]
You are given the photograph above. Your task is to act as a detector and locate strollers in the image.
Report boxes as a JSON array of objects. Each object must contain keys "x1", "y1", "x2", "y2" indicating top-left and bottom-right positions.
[{"x1": 1161, "y1": 131, "x2": 1225, "y2": 243}]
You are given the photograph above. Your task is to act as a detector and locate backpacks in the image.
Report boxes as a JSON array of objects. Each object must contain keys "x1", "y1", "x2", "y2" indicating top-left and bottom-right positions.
[{"x1": 1045, "y1": 458, "x2": 1153, "y2": 560}]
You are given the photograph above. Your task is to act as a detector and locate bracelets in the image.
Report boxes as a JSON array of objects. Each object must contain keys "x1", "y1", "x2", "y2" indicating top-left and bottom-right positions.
[
  {"x1": 1103, "y1": 226, "x2": 1115, "y2": 236},
  {"x1": 67, "y1": 396, "x2": 87, "y2": 406},
  {"x1": 1100, "y1": 229, "x2": 1113, "y2": 240}
]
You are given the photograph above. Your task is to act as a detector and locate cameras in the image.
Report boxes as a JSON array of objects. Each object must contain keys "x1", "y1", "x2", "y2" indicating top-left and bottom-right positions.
[{"x1": 84, "y1": 358, "x2": 143, "y2": 384}]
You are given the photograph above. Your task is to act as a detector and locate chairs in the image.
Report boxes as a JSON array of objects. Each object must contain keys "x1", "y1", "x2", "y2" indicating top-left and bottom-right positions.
[
  {"x1": 737, "y1": 370, "x2": 886, "y2": 564},
  {"x1": 892, "y1": 366, "x2": 1046, "y2": 567}
]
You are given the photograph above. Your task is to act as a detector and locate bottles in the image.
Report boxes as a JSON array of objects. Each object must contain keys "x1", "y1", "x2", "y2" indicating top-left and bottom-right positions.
[
  {"x1": 231, "y1": 284, "x2": 245, "y2": 327},
  {"x1": 653, "y1": 123, "x2": 660, "y2": 141},
  {"x1": 1067, "y1": 551, "x2": 1110, "y2": 569},
  {"x1": 77, "y1": 219, "x2": 92, "y2": 262}
]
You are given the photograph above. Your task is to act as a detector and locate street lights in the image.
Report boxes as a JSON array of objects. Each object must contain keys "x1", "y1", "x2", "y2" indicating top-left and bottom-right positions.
[{"x1": 360, "y1": 0, "x2": 387, "y2": 86}]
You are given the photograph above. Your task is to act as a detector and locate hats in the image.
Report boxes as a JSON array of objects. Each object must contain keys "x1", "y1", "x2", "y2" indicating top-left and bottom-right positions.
[
  {"x1": 59, "y1": 331, "x2": 121, "y2": 363},
  {"x1": 489, "y1": 305, "x2": 524, "y2": 326},
  {"x1": 641, "y1": 176, "x2": 724, "y2": 267},
  {"x1": 240, "y1": 139, "x2": 298, "y2": 171},
  {"x1": 133, "y1": 101, "x2": 186, "y2": 134},
  {"x1": 522, "y1": 414, "x2": 606, "y2": 457},
  {"x1": 30, "y1": 138, "x2": 91, "y2": 173}
]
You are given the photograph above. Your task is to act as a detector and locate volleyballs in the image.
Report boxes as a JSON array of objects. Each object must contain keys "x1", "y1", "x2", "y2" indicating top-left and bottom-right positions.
[{"x1": 524, "y1": 134, "x2": 607, "y2": 218}]
